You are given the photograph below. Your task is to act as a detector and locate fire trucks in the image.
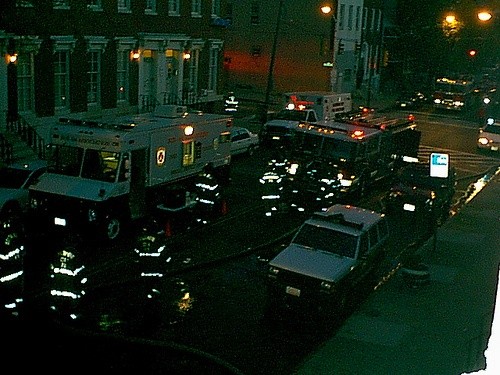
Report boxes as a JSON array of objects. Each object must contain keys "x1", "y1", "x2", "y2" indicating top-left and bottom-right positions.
[
  {"x1": 431, "y1": 76, "x2": 477, "y2": 113},
  {"x1": 266, "y1": 106, "x2": 423, "y2": 204}
]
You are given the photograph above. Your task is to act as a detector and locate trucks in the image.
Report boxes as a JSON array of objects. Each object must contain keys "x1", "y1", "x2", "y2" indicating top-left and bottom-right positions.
[{"x1": 261, "y1": 90, "x2": 354, "y2": 148}]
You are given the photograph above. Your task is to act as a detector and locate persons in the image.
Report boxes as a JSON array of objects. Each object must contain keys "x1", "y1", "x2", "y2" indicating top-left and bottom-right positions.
[
  {"x1": 190, "y1": 161, "x2": 220, "y2": 233},
  {"x1": 222, "y1": 91, "x2": 240, "y2": 121},
  {"x1": 0, "y1": 208, "x2": 30, "y2": 325},
  {"x1": 257, "y1": 153, "x2": 361, "y2": 222},
  {"x1": 130, "y1": 219, "x2": 175, "y2": 315},
  {"x1": 46, "y1": 246, "x2": 92, "y2": 339}
]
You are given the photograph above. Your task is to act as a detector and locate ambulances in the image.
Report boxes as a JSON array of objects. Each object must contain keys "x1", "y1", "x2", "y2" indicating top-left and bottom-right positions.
[{"x1": 29, "y1": 104, "x2": 235, "y2": 244}]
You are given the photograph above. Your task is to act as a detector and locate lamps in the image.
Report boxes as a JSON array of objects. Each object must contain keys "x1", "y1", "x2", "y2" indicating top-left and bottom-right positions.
[
  {"x1": 476, "y1": 9, "x2": 494, "y2": 23},
  {"x1": 445, "y1": 11, "x2": 456, "y2": 23},
  {"x1": 6, "y1": 51, "x2": 17, "y2": 63},
  {"x1": 319, "y1": 2, "x2": 333, "y2": 14},
  {"x1": 133, "y1": 50, "x2": 140, "y2": 59},
  {"x1": 183, "y1": 50, "x2": 190, "y2": 59}
]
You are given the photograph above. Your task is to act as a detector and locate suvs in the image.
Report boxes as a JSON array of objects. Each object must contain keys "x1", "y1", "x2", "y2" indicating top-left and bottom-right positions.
[
  {"x1": 381, "y1": 162, "x2": 459, "y2": 223},
  {"x1": 265, "y1": 204, "x2": 389, "y2": 310}
]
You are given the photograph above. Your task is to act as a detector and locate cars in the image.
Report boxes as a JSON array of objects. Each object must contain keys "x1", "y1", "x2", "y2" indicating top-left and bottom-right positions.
[
  {"x1": 231, "y1": 126, "x2": 260, "y2": 156},
  {"x1": 0, "y1": 156, "x2": 56, "y2": 223},
  {"x1": 475, "y1": 117, "x2": 500, "y2": 155},
  {"x1": 395, "y1": 88, "x2": 431, "y2": 110}
]
